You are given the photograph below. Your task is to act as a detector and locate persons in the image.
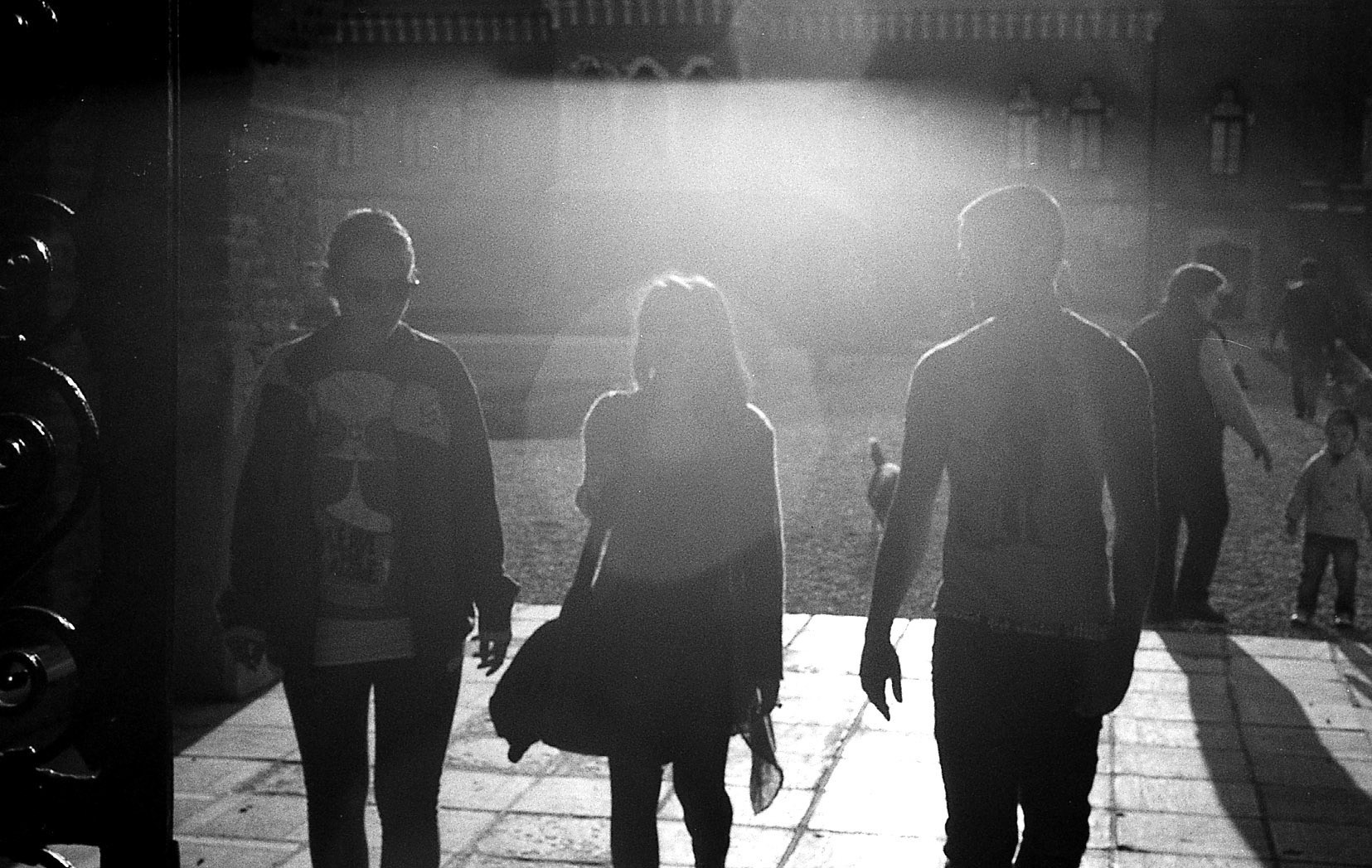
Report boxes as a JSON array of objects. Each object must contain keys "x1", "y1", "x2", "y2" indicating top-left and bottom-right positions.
[
  {"x1": 219, "y1": 209, "x2": 519, "y2": 868},
  {"x1": 1282, "y1": 408, "x2": 1372, "y2": 629},
  {"x1": 1269, "y1": 257, "x2": 1340, "y2": 419},
  {"x1": 1126, "y1": 261, "x2": 1274, "y2": 630},
  {"x1": 573, "y1": 274, "x2": 783, "y2": 868},
  {"x1": 857, "y1": 186, "x2": 1150, "y2": 868}
]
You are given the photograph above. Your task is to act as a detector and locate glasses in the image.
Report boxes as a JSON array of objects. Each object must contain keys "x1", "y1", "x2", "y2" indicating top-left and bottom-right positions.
[{"x1": 339, "y1": 275, "x2": 420, "y2": 300}]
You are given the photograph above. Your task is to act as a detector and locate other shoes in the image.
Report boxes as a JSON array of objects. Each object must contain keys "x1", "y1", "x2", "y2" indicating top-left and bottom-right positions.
[
  {"x1": 1307, "y1": 406, "x2": 1317, "y2": 417},
  {"x1": 1334, "y1": 610, "x2": 1354, "y2": 627},
  {"x1": 1171, "y1": 599, "x2": 1231, "y2": 624},
  {"x1": 1295, "y1": 409, "x2": 1304, "y2": 418},
  {"x1": 1289, "y1": 608, "x2": 1315, "y2": 628},
  {"x1": 1146, "y1": 606, "x2": 1177, "y2": 622}
]
